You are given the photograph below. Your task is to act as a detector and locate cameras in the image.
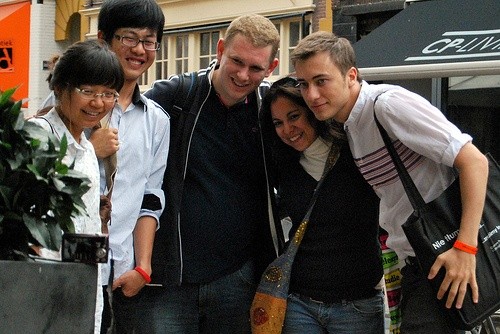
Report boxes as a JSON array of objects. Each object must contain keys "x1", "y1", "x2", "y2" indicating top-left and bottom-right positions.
[{"x1": 62, "y1": 233, "x2": 109, "y2": 264}]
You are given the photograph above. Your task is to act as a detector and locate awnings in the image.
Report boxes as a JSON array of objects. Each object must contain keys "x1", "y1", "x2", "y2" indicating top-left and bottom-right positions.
[{"x1": 343, "y1": 0, "x2": 500, "y2": 82}]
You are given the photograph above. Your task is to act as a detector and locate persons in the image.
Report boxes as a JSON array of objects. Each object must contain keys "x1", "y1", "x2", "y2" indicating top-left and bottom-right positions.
[
  {"x1": 291, "y1": 24, "x2": 500, "y2": 333},
  {"x1": 12, "y1": 38, "x2": 127, "y2": 333},
  {"x1": 30, "y1": 1, "x2": 172, "y2": 333},
  {"x1": 137, "y1": 12, "x2": 289, "y2": 333},
  {"x1": 266, "y1": 67, "x2": 391, "y2": 333}
]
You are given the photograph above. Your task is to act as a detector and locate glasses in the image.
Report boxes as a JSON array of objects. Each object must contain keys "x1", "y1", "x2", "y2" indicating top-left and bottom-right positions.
[
  {"x1": 113, "y1": 34, "x2": 161, "y2": 51},
  {"x1": 72, "y1": 88, "x2": 120, "y2": 102}
]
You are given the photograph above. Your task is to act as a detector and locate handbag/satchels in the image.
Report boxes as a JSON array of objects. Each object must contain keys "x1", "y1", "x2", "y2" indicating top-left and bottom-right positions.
[
  {"x1": 250, "y1": 254, "x2": 292, "y2": 334},
  {"x1": 374, "y1": 89, "x2": 500, "y2": 330}
]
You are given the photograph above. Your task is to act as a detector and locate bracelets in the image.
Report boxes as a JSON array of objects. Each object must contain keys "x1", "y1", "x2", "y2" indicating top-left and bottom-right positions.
[
  {"x1": 450, "y1": 237, "x2": 479, "y2": 256},
  {"x1": 128, "y1": 260, "x2": 155, "y2": 284}
]
